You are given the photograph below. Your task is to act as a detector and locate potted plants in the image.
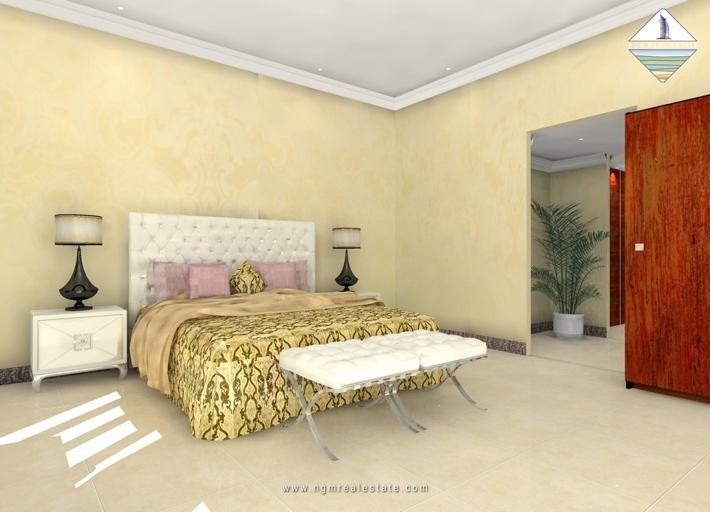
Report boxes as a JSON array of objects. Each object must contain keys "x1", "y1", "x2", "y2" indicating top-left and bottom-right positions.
[{"x1": 530, "y1": 197, "x2": 611, "y2": 338}]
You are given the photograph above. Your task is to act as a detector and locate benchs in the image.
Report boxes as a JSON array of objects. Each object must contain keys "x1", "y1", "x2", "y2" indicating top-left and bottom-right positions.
[{"x1": 278, "y1": 328, "x2": 489, "y2": 462}]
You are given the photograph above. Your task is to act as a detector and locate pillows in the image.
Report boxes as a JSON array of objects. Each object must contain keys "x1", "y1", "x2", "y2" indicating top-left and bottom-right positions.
[{"x1": 154, "y1": 263, "x2": 309, "y2": 298}]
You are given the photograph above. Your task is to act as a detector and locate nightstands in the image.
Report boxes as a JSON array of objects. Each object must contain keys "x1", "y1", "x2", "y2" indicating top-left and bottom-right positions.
[
  {"x1": 344, "y1": 292, "x2": 380, "y2": 298},
  {"x1": 29, "y1": 305, "x2": 129, "y2": 392}
]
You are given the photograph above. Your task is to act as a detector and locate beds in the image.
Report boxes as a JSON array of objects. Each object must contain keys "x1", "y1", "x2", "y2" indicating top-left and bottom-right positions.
[{"x1": 128, "y1": 210, "x2": 447, "y2": 440}]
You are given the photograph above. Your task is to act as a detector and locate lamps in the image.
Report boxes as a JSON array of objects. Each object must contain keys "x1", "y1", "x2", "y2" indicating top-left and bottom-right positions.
[
  {"x1": 331, "y1": 228, "x2": 360, "y2": 292},
  {"x1": 54, "y1": 215, "x2": 103, "y2": 310}
]
